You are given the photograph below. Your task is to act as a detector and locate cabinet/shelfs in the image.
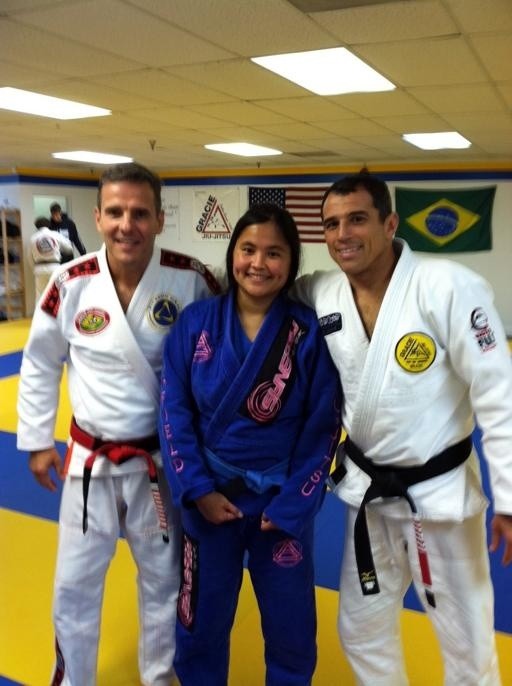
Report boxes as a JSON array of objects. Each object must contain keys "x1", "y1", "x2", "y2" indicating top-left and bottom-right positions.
[{"x1": 1, "y1": 207, "x2": 29, "y2": 325}]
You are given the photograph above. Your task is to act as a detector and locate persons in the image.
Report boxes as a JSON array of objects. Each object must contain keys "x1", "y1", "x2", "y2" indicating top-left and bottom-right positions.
[
  {"x1": 15, "y1": 160, "x2": 221, "y2": 685},
  {"x1": 156, "y1": 203, "x2": 346, "y2": 686},
  {"x1": 288, "y1": 170, "x2": 512, "y2": 686},
  {"x1": 30, "y1": 215, "x2": 74, "y2": 311},
  {"x1": 49, "y1": 200, "x2": 88, "y2": 266}
]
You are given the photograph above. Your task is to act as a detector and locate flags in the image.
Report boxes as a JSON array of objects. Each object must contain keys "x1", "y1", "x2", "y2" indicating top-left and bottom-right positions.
[
  {"x1": 391, "y1": 184, "x2": 497, "y2": 255},
  {"x1": 244, "y1": 185, "x2": 335, "y2": 247}
]
